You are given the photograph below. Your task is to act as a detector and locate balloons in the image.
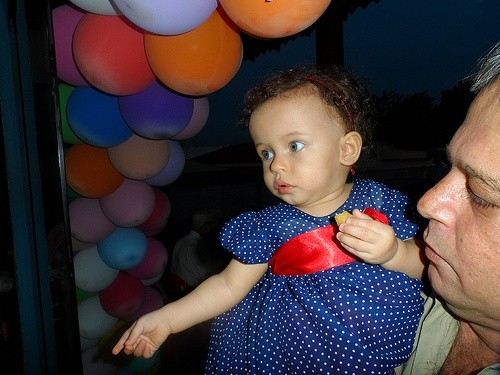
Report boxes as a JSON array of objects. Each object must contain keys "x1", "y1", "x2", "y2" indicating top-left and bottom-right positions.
[{"x1": 51, "y1": 0, "x2": 330, "y2": 375}]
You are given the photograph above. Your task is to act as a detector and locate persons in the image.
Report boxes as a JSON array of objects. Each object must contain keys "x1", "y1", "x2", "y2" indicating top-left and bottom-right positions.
[
  {"x1": 393, "y1": 41, "x2": 500, "y2": 374},
  {"x1": 112, "y1": 63, "x2": 429, "y2": 375}
]
